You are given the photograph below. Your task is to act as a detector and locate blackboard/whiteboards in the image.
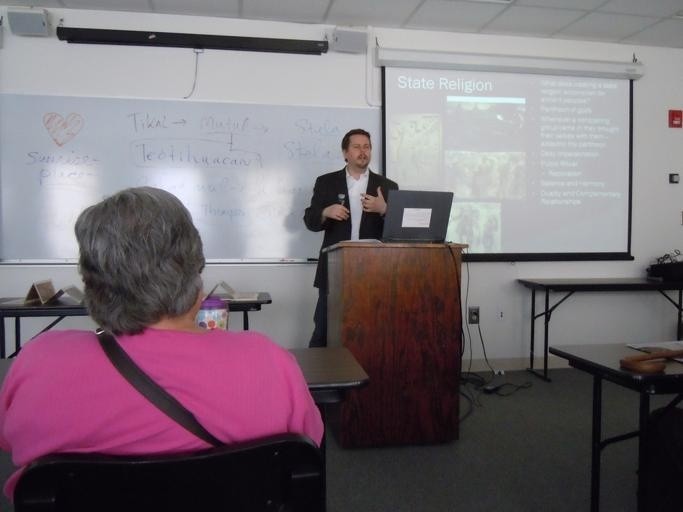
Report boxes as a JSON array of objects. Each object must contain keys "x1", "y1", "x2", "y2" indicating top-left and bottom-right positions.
[{"x1": 0, "y1": 93, "x2": 385, "y2": 266}]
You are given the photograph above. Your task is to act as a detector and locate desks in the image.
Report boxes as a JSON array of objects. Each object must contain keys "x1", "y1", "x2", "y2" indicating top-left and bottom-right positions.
[
  {"x1": 516, "y1": 275, "x2": 682, "y2": 510},
  {"x1": 1, "y1": 289, "x2": 273, "y2": 358},
  {"x1": 1, "y1": 346, "x2": 365, "y2": 433}
]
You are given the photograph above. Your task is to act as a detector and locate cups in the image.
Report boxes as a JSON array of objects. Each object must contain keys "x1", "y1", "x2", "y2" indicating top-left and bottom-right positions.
[{"x1": 194, "y1": 297, "x2": 228, "y2": 333}]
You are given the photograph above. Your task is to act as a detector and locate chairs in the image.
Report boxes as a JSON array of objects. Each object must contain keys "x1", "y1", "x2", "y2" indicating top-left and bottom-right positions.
[{"x1": 14, "y1": 433, "x2": 327, "y2": 510}]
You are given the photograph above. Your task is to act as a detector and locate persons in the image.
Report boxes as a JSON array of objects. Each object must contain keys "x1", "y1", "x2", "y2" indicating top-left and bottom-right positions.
[
  {"x1": 302, "y1": 128, "x2": 401, "y2": 346},
  {"x1": 0, "y1": 186, "x2": 331, "y2": 512}
]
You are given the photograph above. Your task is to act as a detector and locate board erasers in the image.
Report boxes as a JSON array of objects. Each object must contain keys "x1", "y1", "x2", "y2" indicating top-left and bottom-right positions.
[{"x1": 307, "y1": 258, "x2": 319, "y2": 261}]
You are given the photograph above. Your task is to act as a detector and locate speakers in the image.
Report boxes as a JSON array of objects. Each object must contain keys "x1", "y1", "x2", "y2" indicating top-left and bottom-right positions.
[
  {"x1": 332, "y1": 28, "x2": 368, "y2": 54},
  {"x1": 7, "y1": 7, "x2": 50, "y2": 37}
]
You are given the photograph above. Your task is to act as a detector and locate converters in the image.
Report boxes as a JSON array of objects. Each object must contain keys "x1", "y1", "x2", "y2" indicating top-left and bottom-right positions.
[{"x1": 482, "y1": 382, "x2": 504, "y2": 394}]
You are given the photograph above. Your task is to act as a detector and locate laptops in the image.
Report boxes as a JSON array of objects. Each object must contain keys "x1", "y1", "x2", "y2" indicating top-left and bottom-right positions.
[{"x1": 380, "y1": 189, "x2": 454, "y2": 243}]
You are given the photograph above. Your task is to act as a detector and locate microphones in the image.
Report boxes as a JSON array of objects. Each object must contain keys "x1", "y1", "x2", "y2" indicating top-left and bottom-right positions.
[{"x1": 337, "y1": 194, "x2": 346, "y2": 205}]
